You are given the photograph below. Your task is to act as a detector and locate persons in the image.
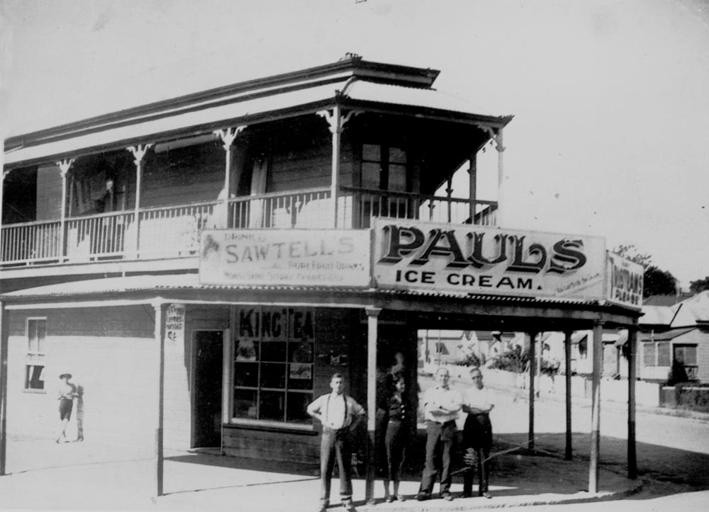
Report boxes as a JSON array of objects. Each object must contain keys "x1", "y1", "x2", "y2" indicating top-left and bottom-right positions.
[
  {"x1": 54, "y1": 373, "x2": 80, "y2": 445},
  {"x1": 305, "y1": 372, "x2": 367, "y2": 512},
  {"x1": 379, "y1": 370, "x2": 417, "y2": 502},
  {"x1": 416, "y1": 367, "x2": 463, "y2": 501},
  {"x1": 460, "y1": 368, "x2": 496, "y2": 498}
]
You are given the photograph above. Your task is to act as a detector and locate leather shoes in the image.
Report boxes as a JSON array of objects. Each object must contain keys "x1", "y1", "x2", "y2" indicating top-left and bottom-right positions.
[{"x1": 318, "y1": 491, "x2": 493, "y2": 511}]
